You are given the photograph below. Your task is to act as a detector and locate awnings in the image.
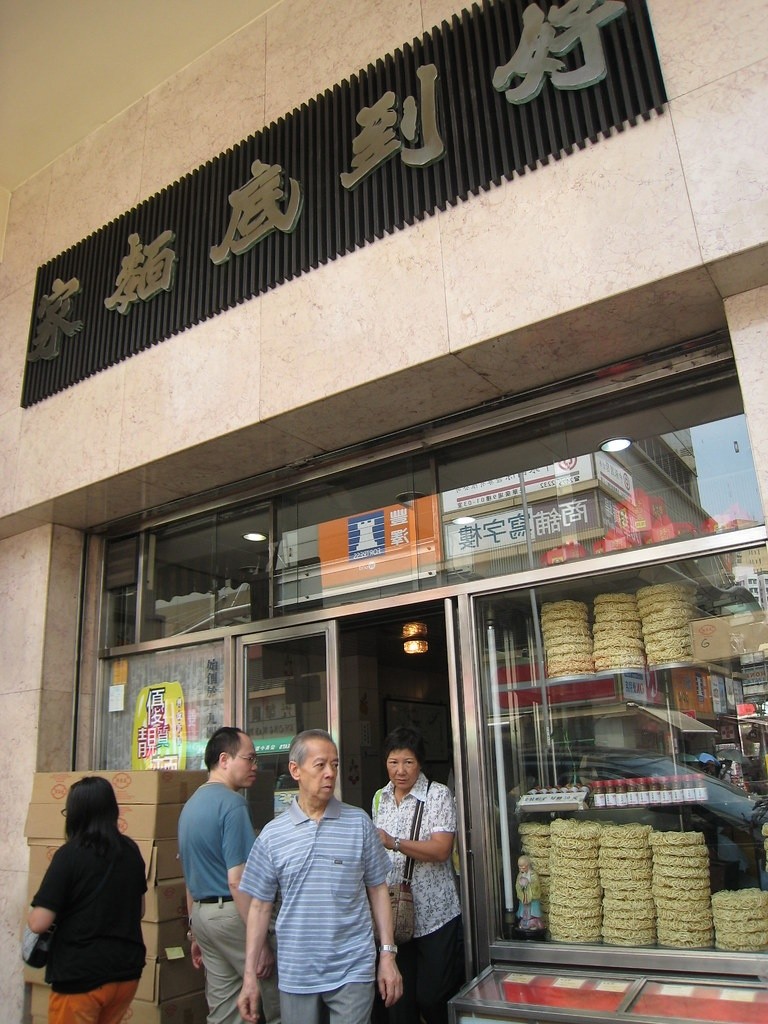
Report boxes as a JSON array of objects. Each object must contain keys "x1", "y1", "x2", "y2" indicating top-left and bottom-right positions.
[{"x1": 642, "y1": 705, "x2": 718, "y2": 734}]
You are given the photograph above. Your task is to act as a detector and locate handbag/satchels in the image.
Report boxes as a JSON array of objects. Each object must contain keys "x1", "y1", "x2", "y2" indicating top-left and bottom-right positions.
[
  {"x1": 366, "y1": 883, "x2": 415, "y2": 941},
  {"x1": 21, "y1": 921, "x2": 54, "y2": 968}
]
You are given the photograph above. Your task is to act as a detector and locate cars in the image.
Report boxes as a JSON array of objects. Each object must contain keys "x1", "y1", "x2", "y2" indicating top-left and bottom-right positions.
[{"x1": 516, "y1": 746, "x2": 767, "y2": 891}]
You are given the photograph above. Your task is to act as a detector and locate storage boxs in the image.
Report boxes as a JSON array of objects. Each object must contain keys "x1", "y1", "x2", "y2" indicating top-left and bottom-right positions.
[
  {"x1": 489, "y1": 609, "x2": 768, "y2": 972},
  {"x1": 22, "y1": 627, "x2": 455, "y2": 1024},
  {"x1": 690, "y1": 610, "x2": 768, "y2": 662}
]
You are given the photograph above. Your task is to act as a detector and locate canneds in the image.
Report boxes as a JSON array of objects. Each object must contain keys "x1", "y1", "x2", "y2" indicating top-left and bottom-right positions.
[{"x1": 526, "y1": 774, "x2": 709, "y2": 809}]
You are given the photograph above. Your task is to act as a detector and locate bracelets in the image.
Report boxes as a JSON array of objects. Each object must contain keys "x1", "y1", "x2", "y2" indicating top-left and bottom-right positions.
[
  {"x1": 378, "y1": 945, "x2": 398, "y2": 953},
  {"x1": 394, "y1": 836, "x2": 400, "y2": 852}
]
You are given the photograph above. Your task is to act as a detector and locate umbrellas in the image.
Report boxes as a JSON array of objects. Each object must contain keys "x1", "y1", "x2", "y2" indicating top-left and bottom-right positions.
[
  {"x1": 679, "y1": 753, "x2": 699, "y2": 762},
  {"x1": 715, "y1": 747, "x2": 753, "y2": 765},
  {"x1": 695, "y1": 753, "x2": 721, "y2": 765}
]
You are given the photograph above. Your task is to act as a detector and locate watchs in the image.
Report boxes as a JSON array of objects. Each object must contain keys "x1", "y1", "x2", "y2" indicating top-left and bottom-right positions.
[{"x1": 186, "y1": 931, "x2": 196, "y2": 941}]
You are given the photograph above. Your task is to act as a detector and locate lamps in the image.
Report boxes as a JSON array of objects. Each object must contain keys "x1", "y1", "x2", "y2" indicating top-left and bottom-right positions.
[{"x1": 401, "y1": 622, "x2": 428, "y2": 653}]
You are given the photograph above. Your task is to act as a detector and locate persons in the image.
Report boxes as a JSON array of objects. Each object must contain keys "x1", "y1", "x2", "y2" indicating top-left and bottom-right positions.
[
  {"x1": 26, "y1": 776, "x2": 148, "y2": 1024},
  {"x1": 368, "y1": 723, "x2": 465, "y2": 1024},
  {"x1": 720, "y1": 759, "x2": 736, "y2": 784},
  {"x1": 515, "y1": 856, "x2": 545, "y2": 930},
  {"x1": 177, "y1": 727, "x2": 282, "y2": 1024},
  {"x1": 236, "y1": 729, "x2": 404, "y2": 1024}
]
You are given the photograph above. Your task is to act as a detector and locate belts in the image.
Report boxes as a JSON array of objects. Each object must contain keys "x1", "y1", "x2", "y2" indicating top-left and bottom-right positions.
[{"x1": 193, "y1": 895, "x2": 234, "y2": 903}]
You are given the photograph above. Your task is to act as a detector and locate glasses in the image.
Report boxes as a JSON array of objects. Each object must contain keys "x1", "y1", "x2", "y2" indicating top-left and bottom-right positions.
[
  {"x1": 61, "y1": 808, "x2": 69, "y2": 817},
  {"x1": 232, "y1": 752, "x2": 258, "y2": 766}
]
